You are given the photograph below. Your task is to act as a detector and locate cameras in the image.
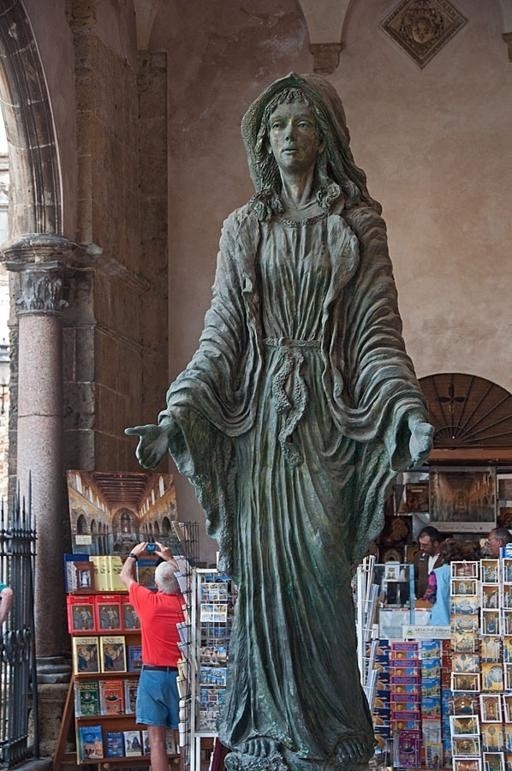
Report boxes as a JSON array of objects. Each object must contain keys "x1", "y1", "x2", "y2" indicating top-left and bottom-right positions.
[{"x1": 145, "y1": 543, "x2": 158, "y2": 552}]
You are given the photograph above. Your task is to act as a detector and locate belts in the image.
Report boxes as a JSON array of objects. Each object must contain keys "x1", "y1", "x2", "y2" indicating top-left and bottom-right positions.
[{"x1": 144, "y1": 665, "x2": 178, "y2": 672}]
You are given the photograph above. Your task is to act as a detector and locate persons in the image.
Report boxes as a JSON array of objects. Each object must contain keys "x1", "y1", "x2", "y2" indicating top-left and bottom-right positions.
[
  {"x1": 122, "y1": 68, "x2": 438, "y2": 771},
  {"x1": 406, "y1": 524, "x2": 512, "y2": 627},
  {"x1": 0, "y1": 582, "x2": 13, "y2": 625}
]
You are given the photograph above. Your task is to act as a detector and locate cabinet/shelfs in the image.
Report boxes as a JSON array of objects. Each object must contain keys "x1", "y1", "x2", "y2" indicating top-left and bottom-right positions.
[
  {"x1": 53, "y1": 542, "x2": 233, "y2": 771},
  {"x1": 353, "y1": 543, "x2": 511, "y2": 770}
]
